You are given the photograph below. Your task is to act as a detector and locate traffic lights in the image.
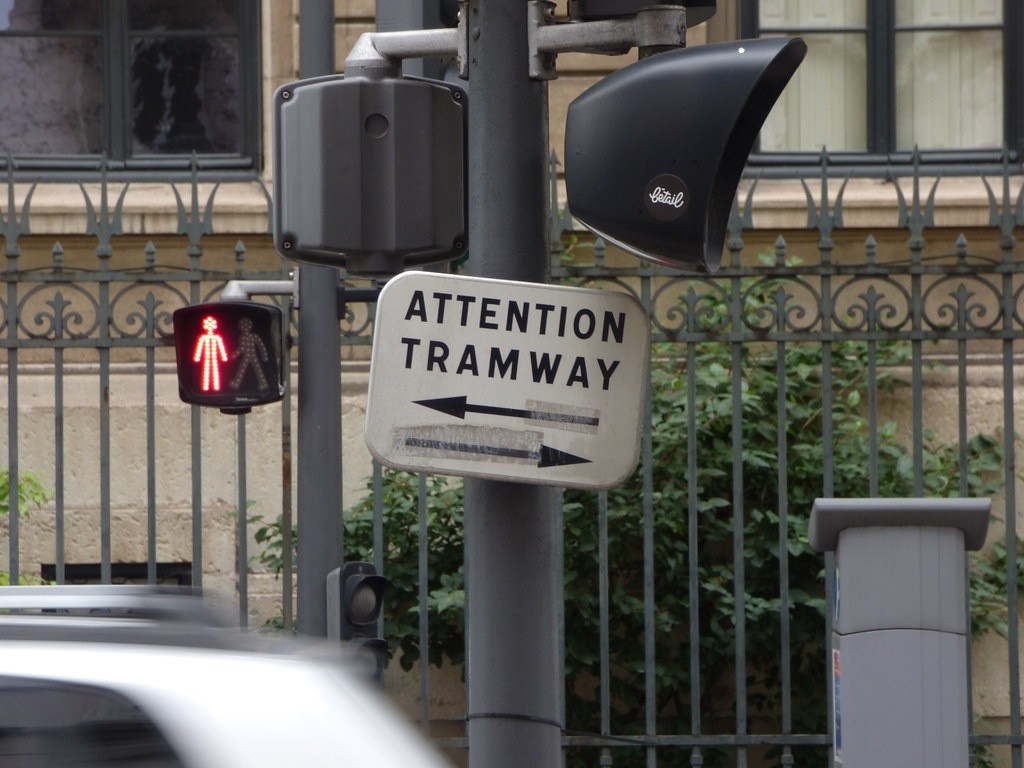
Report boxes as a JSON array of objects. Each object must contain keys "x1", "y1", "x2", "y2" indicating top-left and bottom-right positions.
[
  {"x1": 174, "y1": 301, "x2": 291, "y2": 417},
  {"x1": 325, "y1": 560, "x2": 388, "y2": 688},
  {"x1": 563, "y1": 36, "x2": 808, "y2": 278}
]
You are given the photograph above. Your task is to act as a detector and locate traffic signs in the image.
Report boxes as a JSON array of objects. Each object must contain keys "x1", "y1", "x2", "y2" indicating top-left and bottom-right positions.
[{"x1": 367, "y1": 270, "x2": 651, "y2": 492}]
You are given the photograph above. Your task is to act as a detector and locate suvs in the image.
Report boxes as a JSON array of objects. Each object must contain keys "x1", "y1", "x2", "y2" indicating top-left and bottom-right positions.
[{"x1": 1, "y1": 584, "x2": 455, "y2": 768}]
